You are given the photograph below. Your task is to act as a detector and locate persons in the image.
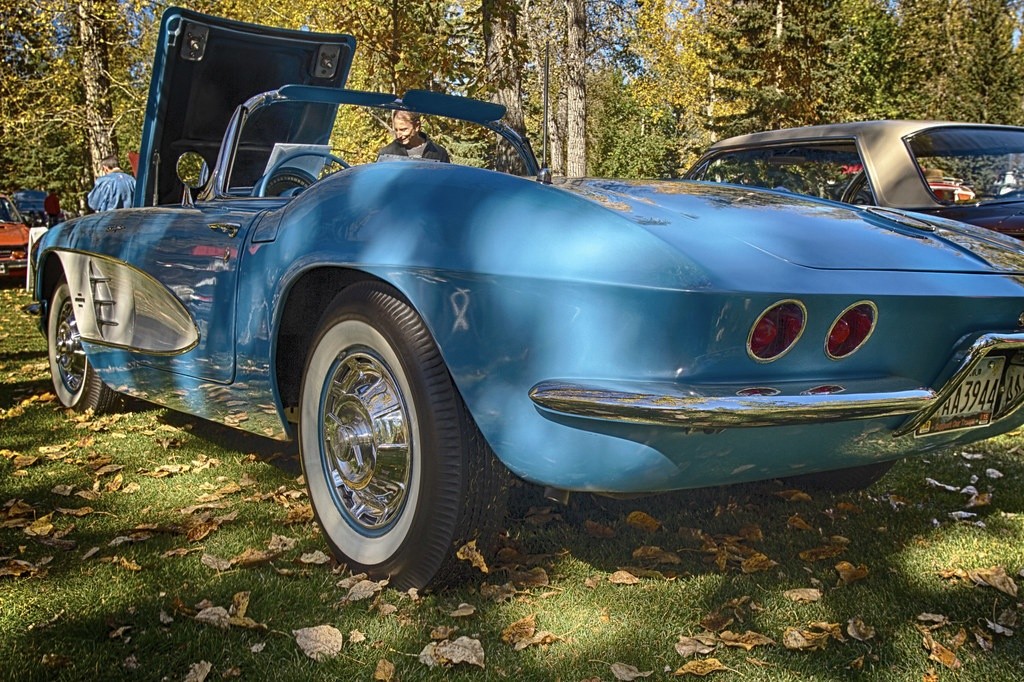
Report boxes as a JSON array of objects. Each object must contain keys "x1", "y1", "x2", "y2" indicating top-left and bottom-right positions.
[
  {"x1": 375, "y1": 108, "x2": 451, "y2": 164},
  {"x1": 44, "y1": 188, "x2": 60, "y2": 229},
  {"x1": 86, "y1": 156, "x2": 137, "y2": 212}
]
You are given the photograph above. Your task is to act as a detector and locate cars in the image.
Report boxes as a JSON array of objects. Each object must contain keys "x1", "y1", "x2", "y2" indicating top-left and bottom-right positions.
[
  {"x1": 13, "y1": 190, "x2": 53, "y2": 227},
  {"x1": 684, "y1": 117, "x2": 1024, "y2": 242},
  {"x1": 0, "y1": 193, "x2": 55, "y2": 285},
  {"x1": 29, "y1": 4, "x2": 1024, "y2": 593}
]
